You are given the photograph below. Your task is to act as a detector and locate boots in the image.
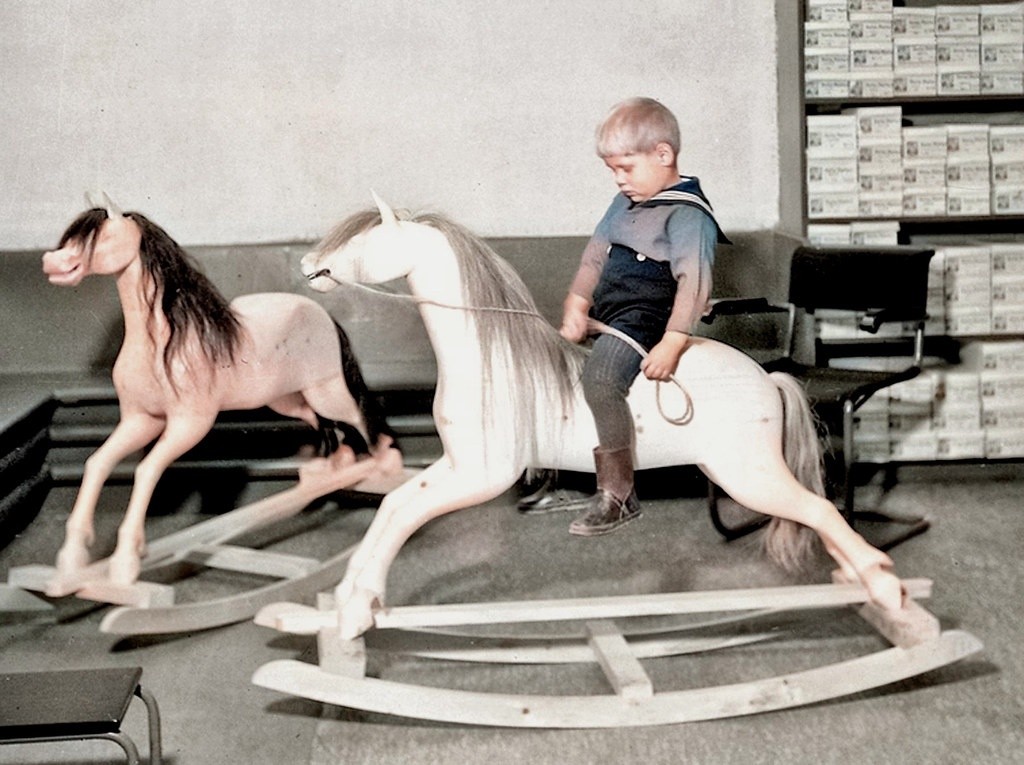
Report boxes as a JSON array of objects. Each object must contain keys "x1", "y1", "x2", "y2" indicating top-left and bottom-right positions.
[
  {"x1": 518, "y1": 469, "x2": 596, "y2": 515},
  {"x1": 568, "y1": 443, "x2": 642, "y2": 538}
]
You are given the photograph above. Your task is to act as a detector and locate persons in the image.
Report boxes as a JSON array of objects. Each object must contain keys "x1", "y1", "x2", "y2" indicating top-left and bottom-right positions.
[{"x1": 553, "y1": 98, "x2": 735, "y2": 538}]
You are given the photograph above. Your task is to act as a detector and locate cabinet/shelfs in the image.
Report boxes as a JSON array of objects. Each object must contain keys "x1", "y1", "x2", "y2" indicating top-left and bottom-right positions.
[{"x1": 776, "y1": 2, "x2": 1024, "y2": 483}]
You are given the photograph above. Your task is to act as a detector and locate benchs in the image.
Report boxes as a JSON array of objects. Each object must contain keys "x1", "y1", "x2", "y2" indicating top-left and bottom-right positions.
[{"x1": 0, "y1": 236, "x2": 788, "y2": 557}]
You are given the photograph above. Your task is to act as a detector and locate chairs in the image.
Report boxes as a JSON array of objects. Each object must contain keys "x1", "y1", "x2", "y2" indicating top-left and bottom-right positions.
[
  {"x1": 2, "y1": 667, "x2": 162, "y2": 764},
  {"x1": 701, "y1": 242, "x2": 934, "y2": 553}
]
